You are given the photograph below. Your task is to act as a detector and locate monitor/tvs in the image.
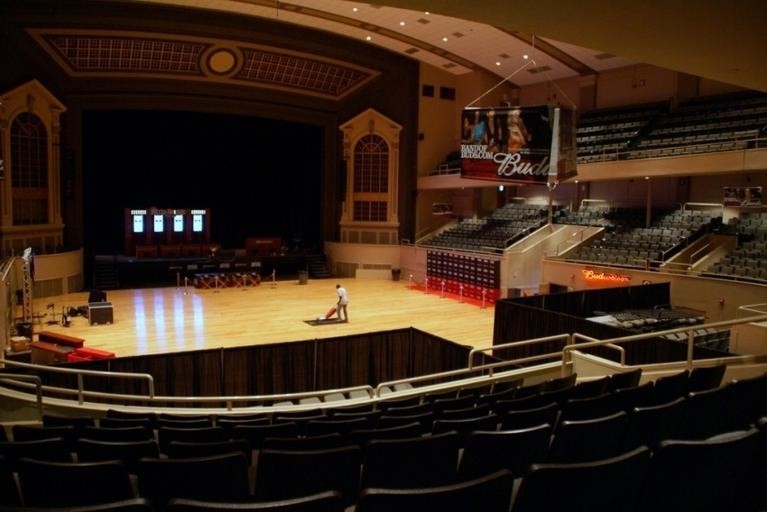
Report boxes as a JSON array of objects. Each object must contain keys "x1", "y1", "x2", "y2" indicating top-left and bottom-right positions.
[
  {"x1": 154, "y1": 215, "x2": 163, "y2": 232},
  {"x1": 174, "y1": 215, "x2": 184, "y2": 232},
  {"x1": 193, "y1": 215, "x2": 203, "y2": 232},
  {"x1": 133, "y1": 215, "x2": 144, "y2": 233}
]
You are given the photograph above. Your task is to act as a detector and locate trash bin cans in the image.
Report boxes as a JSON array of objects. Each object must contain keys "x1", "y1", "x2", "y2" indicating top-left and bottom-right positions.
[
  {"x1": 298, "y1": 270, "x2": 309, "y2": 285},
  {"x1": 508, "y1": 288, "x2": 521, "y2": 299},
  {"x1": 392, "y1": 269, "x2": 400, "y2": 280},
  {"x1": 17, "y1": 323, "x2": 32, "y2": 337}
]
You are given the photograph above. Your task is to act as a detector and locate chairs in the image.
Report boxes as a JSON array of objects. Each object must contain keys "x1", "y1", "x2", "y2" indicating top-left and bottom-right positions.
[{"x1": 575, "y1": 97, "x2": 767, "y2": 164}]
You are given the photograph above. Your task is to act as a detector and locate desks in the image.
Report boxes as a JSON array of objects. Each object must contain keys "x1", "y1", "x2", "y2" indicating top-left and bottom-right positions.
[{"x1": 135, "y1": 238, "x2": 281, "y2": 292}]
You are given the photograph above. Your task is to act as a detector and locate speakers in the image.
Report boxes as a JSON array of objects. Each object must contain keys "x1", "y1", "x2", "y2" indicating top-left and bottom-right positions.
[{"x1": 419, "y1": 133, "x2": 424, "y2": 140}]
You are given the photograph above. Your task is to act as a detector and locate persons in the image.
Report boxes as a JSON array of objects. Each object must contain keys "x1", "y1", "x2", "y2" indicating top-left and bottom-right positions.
[
  {"x1": 335, "y1": 284, "x2": 348, "y2": 323},
  {"x1": 462, "y1": 110, "x2": 532, "y2": 157}
]
